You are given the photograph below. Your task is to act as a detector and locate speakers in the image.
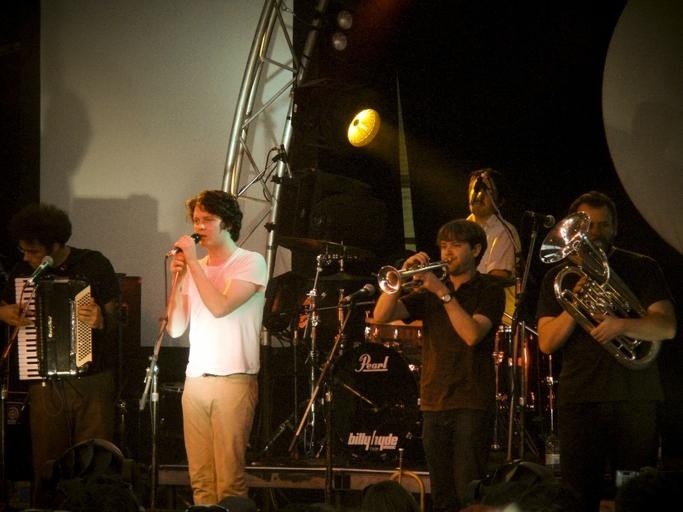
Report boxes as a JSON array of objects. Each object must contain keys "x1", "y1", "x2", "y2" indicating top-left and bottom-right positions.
[{"x1": 112, "y1": 273, "x2": 141, "y2": 466}]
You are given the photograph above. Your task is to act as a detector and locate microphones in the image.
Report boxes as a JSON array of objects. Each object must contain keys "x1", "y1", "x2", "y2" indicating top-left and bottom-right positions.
[
  {"x1": 166, "y1": 234, "x2": 202, "y2": 259},
  {"x1": 339, "y1": 284, "x2": 377, "y2": 304},
  {"x1": 524, "y1": 210, "x2": 555, "y2": 228},
  {"x1": 23, "y1": 254, "x2": 55, "y2": 289}
]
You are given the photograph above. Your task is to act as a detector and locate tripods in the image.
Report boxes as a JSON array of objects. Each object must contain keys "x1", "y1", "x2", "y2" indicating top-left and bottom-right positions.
[{"x1": 252, "y1": 319, "x2": 326, "y2": 465}]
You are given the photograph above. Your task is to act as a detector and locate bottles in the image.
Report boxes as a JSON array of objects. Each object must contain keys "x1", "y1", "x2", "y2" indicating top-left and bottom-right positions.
[{"x1": 545, "y1": 430, "x2": 563, "y2": 480}]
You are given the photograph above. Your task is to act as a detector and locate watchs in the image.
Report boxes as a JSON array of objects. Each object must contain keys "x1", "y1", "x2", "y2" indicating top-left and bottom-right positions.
[{"x1": 439, "y1": 293, "x2": 453, "y2": 304}]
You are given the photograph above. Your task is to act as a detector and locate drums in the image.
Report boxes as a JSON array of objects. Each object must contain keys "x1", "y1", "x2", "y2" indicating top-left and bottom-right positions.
[
  {"x1": 364, "y1": 320, "x2": 424, "y2": 351},
  {"x1": 496, "y1": 325, "x2": 528, "y2": 409},
  {"x1": 319, "y1": 343, "x2": 423, "y2": 462}
]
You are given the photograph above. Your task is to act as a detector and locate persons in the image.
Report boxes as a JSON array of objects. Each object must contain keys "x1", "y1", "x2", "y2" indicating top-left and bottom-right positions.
[
  {"x1": 464, "y1": 167, "x2": 522, "y2": 344},
  {"x1": 0, "y1": 202, "x2": 123, "y2": 511},
  {"x1": 56, "y1": 467, "x2": 681, "y2": 511},
  {"x1": 371, "y1": 218, "x2": 506, "y2": 511},
  {"x1": 533, "y1": 189, "x2": 677, "y2": 511},
  {"x1": 165, "y1": 188, "x2": 269, "y2": 508}
]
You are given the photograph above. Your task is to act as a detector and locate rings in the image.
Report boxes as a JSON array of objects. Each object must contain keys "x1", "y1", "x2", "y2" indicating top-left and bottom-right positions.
[{"x1": 85, "y1": 316, "x2": 90, "y2": 320}]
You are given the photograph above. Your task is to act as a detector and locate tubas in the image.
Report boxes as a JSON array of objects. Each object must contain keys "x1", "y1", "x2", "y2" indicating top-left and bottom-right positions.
[{"x1": 539, "y1": 212, "x2": 661, "y2": 372}]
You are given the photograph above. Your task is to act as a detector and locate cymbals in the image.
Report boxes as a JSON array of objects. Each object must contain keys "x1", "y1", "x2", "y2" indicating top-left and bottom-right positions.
[
  {"x1": 278, "y1": 235, "x2": 373, "y2": 261},
  {"x1": 310, "y1": 195, "x2": 388, "y2": 232},
  {"x1": 309, "y1": 273, "x2": 376, "y2": 283}
]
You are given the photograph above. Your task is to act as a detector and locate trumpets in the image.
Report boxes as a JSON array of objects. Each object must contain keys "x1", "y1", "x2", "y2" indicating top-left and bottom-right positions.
[{"x1": 376, "y1": 259, "x2": 452, "y2": 294}]
